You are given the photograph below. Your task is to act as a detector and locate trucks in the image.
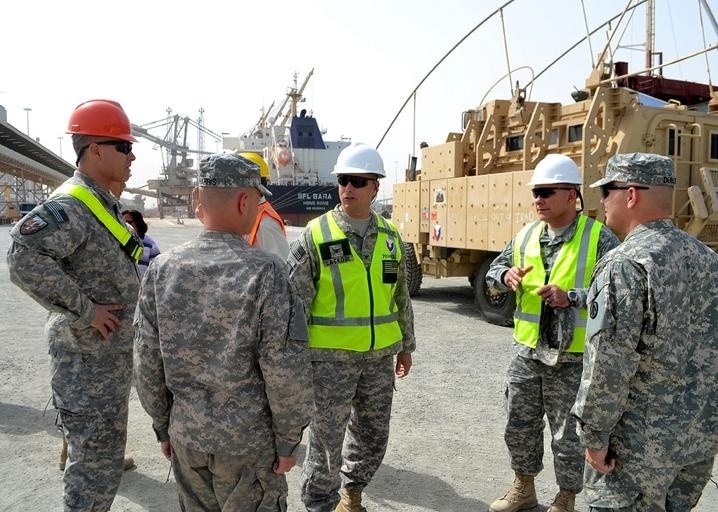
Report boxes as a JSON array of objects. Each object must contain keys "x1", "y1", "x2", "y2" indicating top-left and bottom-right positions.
[{"x1": 19, "y1": 200, "x2": 36, "y2": 217}]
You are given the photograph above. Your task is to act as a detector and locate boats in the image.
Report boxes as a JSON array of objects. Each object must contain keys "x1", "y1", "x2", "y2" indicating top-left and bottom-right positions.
[{"x1": 218, "y1": 66, "x2": 352, "y2": 229}]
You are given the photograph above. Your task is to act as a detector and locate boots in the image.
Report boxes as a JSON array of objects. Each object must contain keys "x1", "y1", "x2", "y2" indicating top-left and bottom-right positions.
[
  {"x1": 334, "y1": 483, "x2": 366, "y2": 512},
  {"x1": 57, "y1": 434, "x2": 134, "y2": 472},
  {"x1": 489, "y1": 472, "x2": 538, "y2": 512},
  {"x1": 547, "y1": 487, "x2": 576, "y2": 512}
]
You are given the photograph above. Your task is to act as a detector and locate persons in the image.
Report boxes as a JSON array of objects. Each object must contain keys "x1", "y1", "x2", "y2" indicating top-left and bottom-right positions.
[
  {"x1": 234, "y1": 151, "x2": 289, "y2": 268},
  {"x1": 5, "y1": 101, "x2": 146, "y2": 511},
  {"x1": 120, "y1": 206, "x2": 159, "y2": 283},
  {"x1": 52, "y1": 180, "x2": 136, "y2": 473},
  {"x1": 567, "y1": 152, "x2": 717, "y2": 511},
  {"x1": 280, "y1": 145, "x2": 415, "y2": 512},
  {"x1": 484, "y1": 153, "x2": 622, "y2": 511},
  {"x1": 129, "y1": 151, "x2": 316, "y2": 510}
]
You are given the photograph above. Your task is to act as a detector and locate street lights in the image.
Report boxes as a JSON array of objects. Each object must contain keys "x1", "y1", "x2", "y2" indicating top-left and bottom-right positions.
[
  {"x1": 57, "y1": 136, "x2": 64, "y2": 156},
  {"x1": 24, "y1": 108, "x2": 33, "y2": 137}
]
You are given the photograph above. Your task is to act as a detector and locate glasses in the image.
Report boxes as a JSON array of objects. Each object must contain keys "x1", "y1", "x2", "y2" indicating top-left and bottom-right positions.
[
  {"x1": 531, "y1": 187, "x2": 571, "y2": 199},
  {"x1": 337, "y1": 173, "x2": 376, "y2": 188},
  {"x1": 598, "y1": 185, "x2": 648, "y2": 198},
  {"x1": 83, "y1": 140, "x2": 133, "y2": 154}
]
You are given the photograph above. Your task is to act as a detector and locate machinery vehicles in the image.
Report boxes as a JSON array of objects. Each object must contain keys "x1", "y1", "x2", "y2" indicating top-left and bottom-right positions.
[{"x1": 367, "y1": 1, "x2": 718, "y2": 328}]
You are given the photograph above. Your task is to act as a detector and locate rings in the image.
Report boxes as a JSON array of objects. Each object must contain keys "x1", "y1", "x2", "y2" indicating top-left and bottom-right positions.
[{"x1": 545, "y1": 298, "x2": 550, "y2": 303}]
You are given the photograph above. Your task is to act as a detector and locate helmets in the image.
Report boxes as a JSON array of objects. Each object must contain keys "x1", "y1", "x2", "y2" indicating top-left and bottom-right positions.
[
  {"x1": 240, "y1": 151, "x2": 270, "y2": 179},
  {"x1": 330, "y1": 142, "x2": 386, "y2": 178},
  {"x1": 528, "y1": 154, "x2": 582, "y2": 187},
  {"x1": 65, "y1": 99, "x2": 138, "y2": 142}
]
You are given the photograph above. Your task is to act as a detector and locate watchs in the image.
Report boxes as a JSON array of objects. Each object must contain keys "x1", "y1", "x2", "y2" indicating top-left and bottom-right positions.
[{"x1": 567, "y1": 288, "x2": 578, "y2": 308}]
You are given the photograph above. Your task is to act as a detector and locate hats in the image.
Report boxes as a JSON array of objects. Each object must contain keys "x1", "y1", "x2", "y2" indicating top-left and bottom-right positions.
[
  {"x1": 588, "y1": 152, "x2": 675, "y2": 190},
  {"x1": 532, "y1": 295, "x2": 576, "y2": 368},
  {"x1": 199, "y1": 152, "x2": 272, "y2": 195}
]
